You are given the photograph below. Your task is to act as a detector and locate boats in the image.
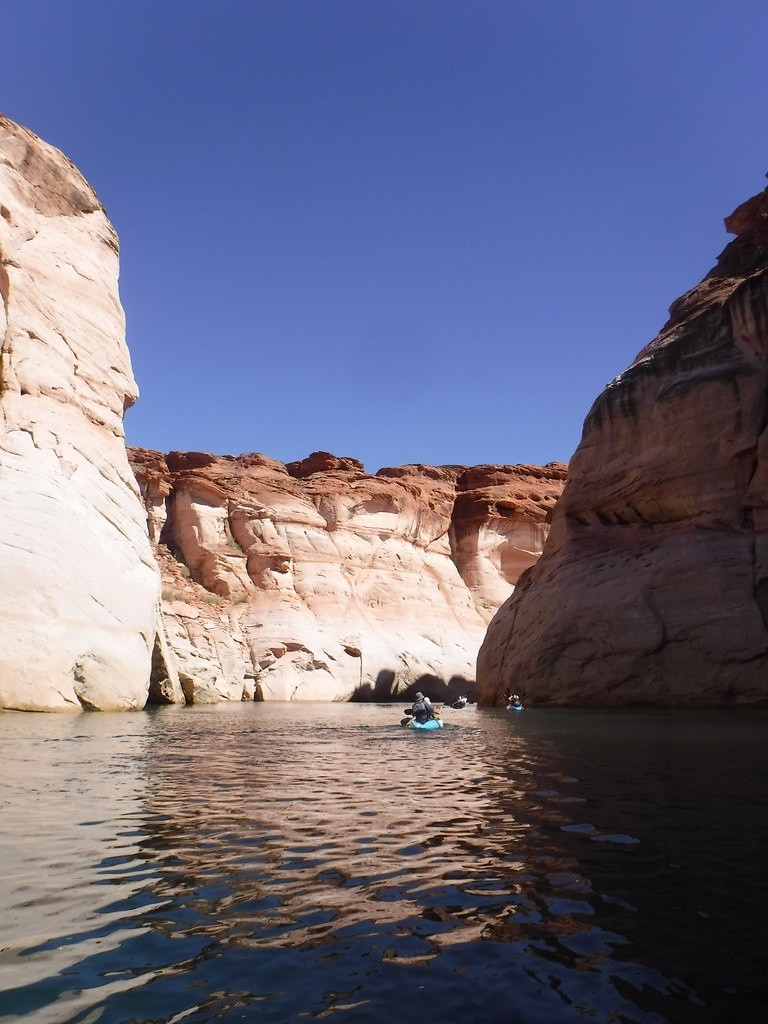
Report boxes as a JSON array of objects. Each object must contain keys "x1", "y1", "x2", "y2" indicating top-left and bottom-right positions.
[
  {"x1": 407, "y1": 716, "x2": 444, "y2": 731},
  {"x1": 506, "y1": 704, "x2": 525, "y2": 711}
]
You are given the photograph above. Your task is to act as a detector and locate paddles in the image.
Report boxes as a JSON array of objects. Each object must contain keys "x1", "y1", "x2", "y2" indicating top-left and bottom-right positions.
[
  {"x1": 400, "y1": 717, "x2": 414, "y2": 726},
  {"x1": 404, "y1": 709, "x2": 413, "y2": 715}
]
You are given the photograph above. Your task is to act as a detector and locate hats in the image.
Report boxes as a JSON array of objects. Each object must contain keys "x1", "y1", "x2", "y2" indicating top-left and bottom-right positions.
[
  {"x1": 423, "y1": 697, "x2": 431, "y2": 704},
  {"x1": 416, "y1": 692, "x2": 424, "y2": 700}
]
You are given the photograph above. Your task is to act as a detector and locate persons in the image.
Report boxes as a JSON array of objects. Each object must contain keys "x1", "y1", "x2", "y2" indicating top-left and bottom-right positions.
[
  {"x1": 411, "y1": 692, "x2": 443, "y2": 724},
  {"x1": 509, "y1": 692, "x2": 520, "y2": 706}
]
